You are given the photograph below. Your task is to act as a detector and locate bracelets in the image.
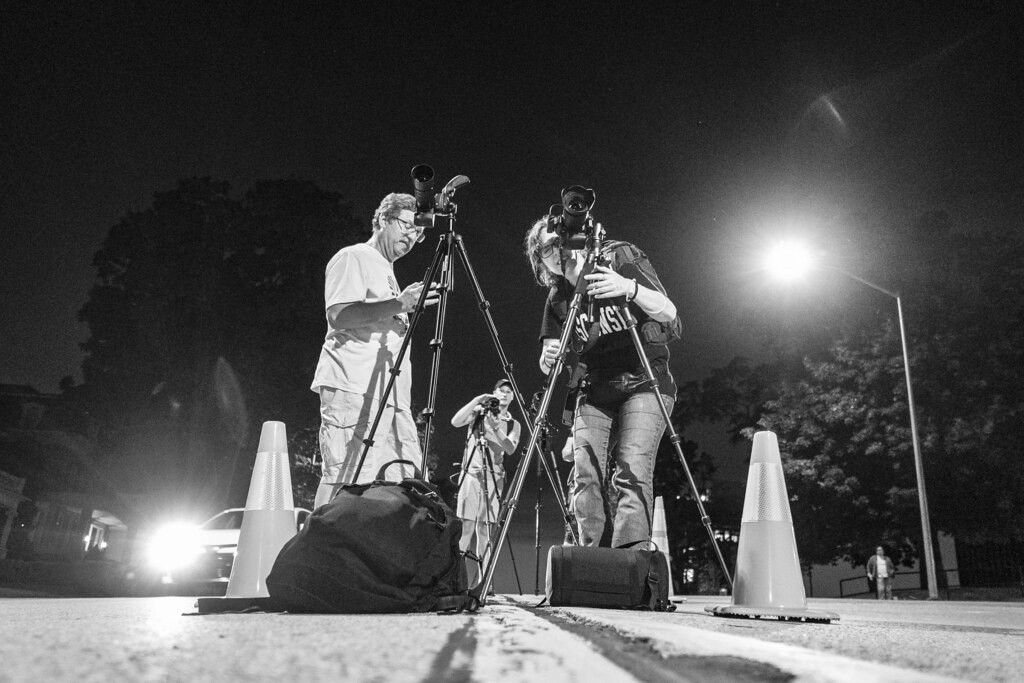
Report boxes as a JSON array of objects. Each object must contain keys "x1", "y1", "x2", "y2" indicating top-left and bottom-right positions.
[
  {"x1": 492, "y1": 427, "x2": 499, "y2": 432},
  {"x1": 630, "y1": 282, "x2": 639, "y2": 301},
  {"x1": 626, "y1": 277, "x2": 636, "y2": 302}
]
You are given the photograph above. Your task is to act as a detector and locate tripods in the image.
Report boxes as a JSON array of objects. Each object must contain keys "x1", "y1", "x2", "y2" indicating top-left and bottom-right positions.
[
  {"x1": 451, "y1": 411, "x2": 522, "y2": 597},
  {"x1": 348, "y1": 210, "x2": 578, "y2": 547},
  {"x1": 475, "y1": 219, "x2": 734, "y2": 607}
]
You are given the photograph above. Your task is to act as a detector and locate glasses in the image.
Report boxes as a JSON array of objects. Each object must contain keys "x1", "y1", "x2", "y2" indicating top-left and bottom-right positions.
[
  {"x1": 393, "y1": 217, "x2": 425, "y2": 243},
  {"x1": 539, "y1": 237, "x2": 559, "y2": 259}
]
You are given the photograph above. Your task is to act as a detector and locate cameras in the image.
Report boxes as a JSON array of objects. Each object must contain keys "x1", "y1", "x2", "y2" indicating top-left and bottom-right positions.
[{"x1": 479, "y1": 397, "x2": 499, "y2": 415}]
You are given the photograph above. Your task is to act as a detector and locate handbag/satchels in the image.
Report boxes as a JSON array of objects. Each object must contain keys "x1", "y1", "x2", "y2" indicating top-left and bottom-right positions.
[
  {"x1": 195, "y1": 458, "x2": 471, "y2": 617},
  {"x1": 545, "y1": 539, "x2": 678, "y2": 613}
]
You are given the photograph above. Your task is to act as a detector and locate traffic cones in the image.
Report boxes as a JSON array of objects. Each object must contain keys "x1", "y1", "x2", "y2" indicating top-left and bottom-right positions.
[
  {"x1": 196, "y1": 421, "x2": 299, "y2": 615},
  {"x1": 704, "y1": 429, "x2": 841, "y2": 624},
  {"x1": 650, "y1": 496, "x2": 687, "y2": 605}
]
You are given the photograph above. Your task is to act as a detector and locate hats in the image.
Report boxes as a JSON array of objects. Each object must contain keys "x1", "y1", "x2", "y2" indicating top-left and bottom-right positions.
[{"x1": 494, "y1": 378, "x2": 513, "y2": 392}]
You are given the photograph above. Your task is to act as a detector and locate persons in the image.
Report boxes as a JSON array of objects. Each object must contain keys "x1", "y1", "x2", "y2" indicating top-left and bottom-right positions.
[
  {"x1": 311, "y1": 193, "x2": 442, "y2": 512},
  {"x1": 450, "y1": 378, "x2": 521, "y2": 595},
  {"x1": 867, "y1": 546, "x2": 895, "y2": 600},
  {"x1": 562, "y1": 417, "x2": 586, "y2": 545},
  {"x1": 526, "y1": 210, "x2": 684, "y2": 550}
]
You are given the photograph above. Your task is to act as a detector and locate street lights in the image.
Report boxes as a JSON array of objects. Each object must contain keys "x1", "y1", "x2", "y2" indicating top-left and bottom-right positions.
[{"x1": 767, "y1": 240, "x2": 939, "y2": 601}]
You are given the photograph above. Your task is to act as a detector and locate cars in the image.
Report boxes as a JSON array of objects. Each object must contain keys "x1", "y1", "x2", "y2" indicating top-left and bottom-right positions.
[{"x1": 171, "y1": 507, "x2": 312, "y2": 588}]
